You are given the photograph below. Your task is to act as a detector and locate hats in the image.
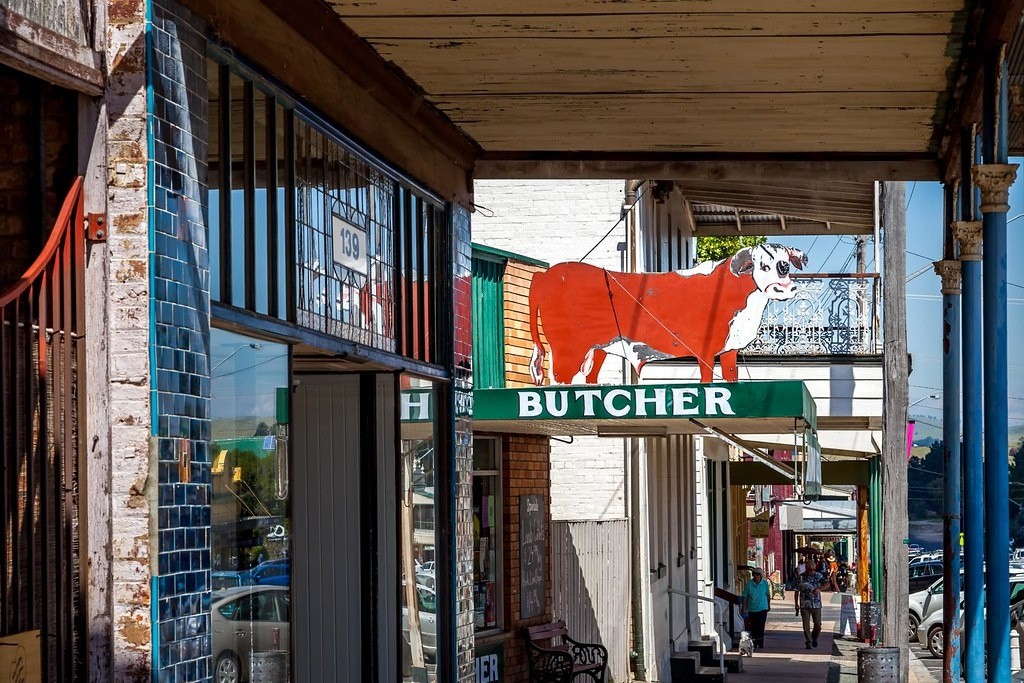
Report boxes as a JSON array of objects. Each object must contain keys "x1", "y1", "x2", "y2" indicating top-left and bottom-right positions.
[
  {"x1": 827, "y1": 556, "x2": 836, "y2": 562},
  {"x1": 753, "y1": 567, "x2": 764, "y2": 578}
]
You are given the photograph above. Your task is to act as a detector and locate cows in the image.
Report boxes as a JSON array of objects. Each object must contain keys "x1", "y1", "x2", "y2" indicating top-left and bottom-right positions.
[{"x1": 529, "y1": 244, "x2": 809, "y2": 386}]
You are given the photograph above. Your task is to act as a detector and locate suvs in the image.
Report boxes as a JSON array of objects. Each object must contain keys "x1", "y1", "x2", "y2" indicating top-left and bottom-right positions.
[
  {"x1": 919, "y1": 577, "x2": 1024, "y2": 657},
  {"x1": 908, "y1": 560, "x2": 943, "y2": 590},
  {"x1": 908, "y1": 568, "x2": 1024, "y2": 650},
  {"x1": 212, "y1": 571, "x2": 256, "y2": 586},
  {"x1": 253, "y1": 564, "x2": 292, "y2": 585}
]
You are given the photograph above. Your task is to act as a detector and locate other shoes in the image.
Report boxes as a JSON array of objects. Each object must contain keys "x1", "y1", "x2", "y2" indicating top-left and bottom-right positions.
[
  {"x1": 758, "y1": 644, "x2": 764, "y2": 649},
  {"x1": 813, "y1": 639, "x2": 819, "y2": 647},
  {"x1": 806, "y1": 644, "x2": 813, "y2": 649}
]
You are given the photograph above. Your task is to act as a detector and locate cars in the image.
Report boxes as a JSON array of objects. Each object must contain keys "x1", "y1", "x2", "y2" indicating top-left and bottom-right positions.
[
  {"x1": 413, "y1": 558, "x2": 434, "y2": 576},
  {"x1": 908, "y1": 544, "x2": 1024, "y2": 562},
  {"x1": 211, "y1": 584, "x2": 290, "y2": 683},
  {"x1": 239, "y1": 559, "x2": 292, "y2": 587},
  {"x1": 416, "y1": 573, "x2": 435, "y2": 588},
  {"x1": 960, "y1": 600, "x2": 1024, "y2": 678},
  {"x1": 402, "y1": 581, "x2": 436, "y2": 664}
]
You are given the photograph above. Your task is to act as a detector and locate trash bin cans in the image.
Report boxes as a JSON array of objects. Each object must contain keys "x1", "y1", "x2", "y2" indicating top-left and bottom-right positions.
[
  {"x1": 248, "y1": 650, "x2": 288, "y2": 683},
  {"x1": 856, "y1": 646, "x2": 900, "y2": 683},
  {"x1": 858, "y1": 602, "x2": 881, "y2": 642}
]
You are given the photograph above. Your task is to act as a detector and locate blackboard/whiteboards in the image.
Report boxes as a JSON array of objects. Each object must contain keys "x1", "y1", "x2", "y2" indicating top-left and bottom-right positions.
[{"x1": 519, "y1": 493, "x2": 546, "y2": 620}]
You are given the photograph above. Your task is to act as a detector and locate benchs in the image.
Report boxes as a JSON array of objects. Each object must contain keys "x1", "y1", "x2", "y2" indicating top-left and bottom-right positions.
[{"x1": 520, "y1": 620, "x2": 609, "y2": 683}]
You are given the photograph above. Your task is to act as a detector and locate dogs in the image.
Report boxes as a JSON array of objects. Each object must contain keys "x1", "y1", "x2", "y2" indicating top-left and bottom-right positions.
[{"x1": 739, "y1": 631, "x2": 754, "y2": 658}]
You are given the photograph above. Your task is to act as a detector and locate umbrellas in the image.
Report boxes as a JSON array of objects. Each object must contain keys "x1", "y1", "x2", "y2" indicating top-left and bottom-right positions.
[{"x1": 794, "y1": 546, "x2": 822, "y2": 557}]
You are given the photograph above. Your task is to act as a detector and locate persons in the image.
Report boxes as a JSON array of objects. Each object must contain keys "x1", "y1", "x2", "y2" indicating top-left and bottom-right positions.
[
  {"x1": 804, "y1": 549, "x2": 857, "y2": 588},
  {"x1": 739, "y1": 595, "x2": 750, "y2": 632},
  {"x1": 827, "y1": 556, "x2": 840, "y2": 593},
  {"x1": 795, "y1": 559, "x2": 806, "y2": 584},
  {"x1": 793, "y1": 561, "x2": 831, "y2": 649},
  {"x1": 741, "y1": 568, "x2": 771, "y2": 649}
]
